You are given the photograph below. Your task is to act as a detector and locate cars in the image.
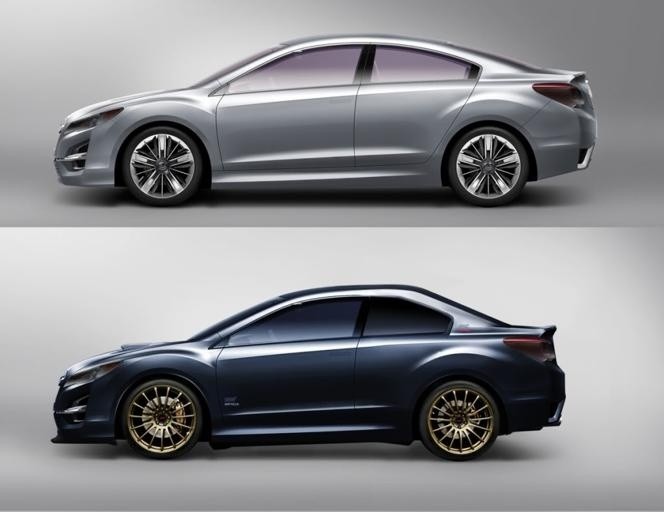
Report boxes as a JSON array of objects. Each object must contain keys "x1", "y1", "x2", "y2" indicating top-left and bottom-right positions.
[
  {"x1": 53, "y1": 31, "x2": 597, "y2": 208},
  {"x1": 50, "y1": 284, "x2": 565, "y2": 462}
]
